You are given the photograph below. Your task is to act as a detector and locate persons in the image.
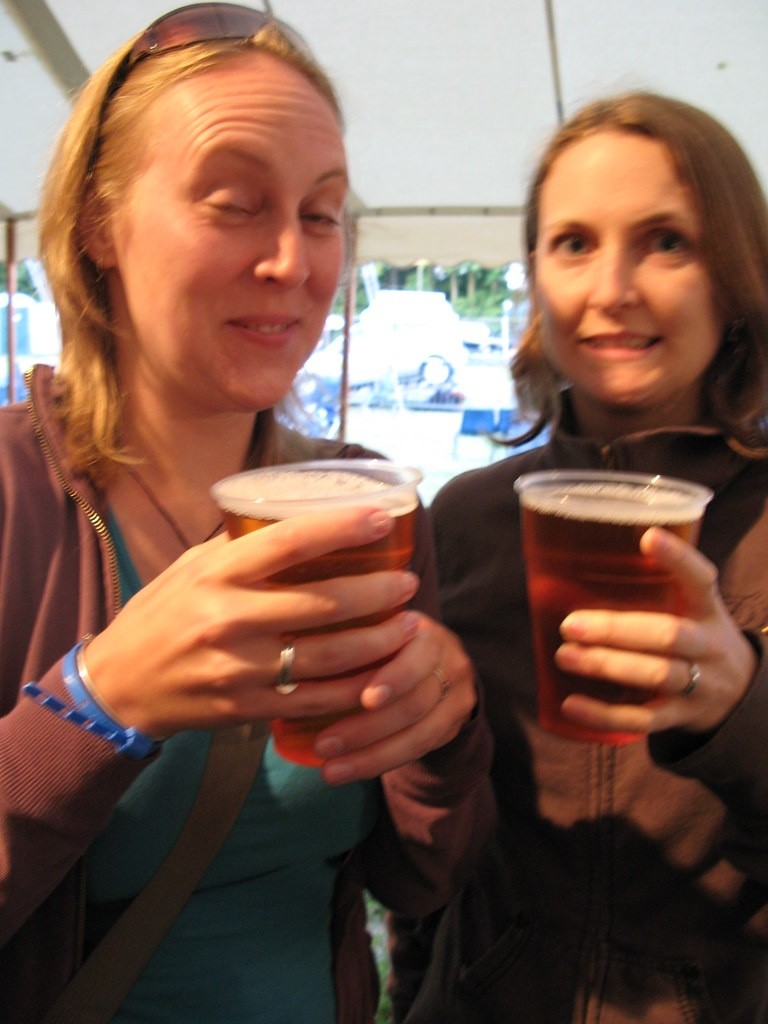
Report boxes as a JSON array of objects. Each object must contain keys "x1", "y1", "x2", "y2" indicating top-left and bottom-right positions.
[
  {"x1": 359, "y1": 87, "x2": 768, "y2": 1024},
  {"x1": 2, "y1": 2, "x2": 496, "y2": 1024}
]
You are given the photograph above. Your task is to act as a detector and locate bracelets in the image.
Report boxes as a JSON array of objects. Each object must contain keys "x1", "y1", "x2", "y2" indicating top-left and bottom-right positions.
[{"x1": 25, "y1": 639, "x2": 164, "y2": 764}]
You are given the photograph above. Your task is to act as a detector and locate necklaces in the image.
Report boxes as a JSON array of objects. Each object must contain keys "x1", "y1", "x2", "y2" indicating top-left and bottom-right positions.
[{"x1": 118, "y1": 447, "x2": 223, "y2": 552}]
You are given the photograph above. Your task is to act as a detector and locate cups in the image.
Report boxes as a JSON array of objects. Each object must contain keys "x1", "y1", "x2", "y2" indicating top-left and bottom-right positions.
[
  {"x1": 209, "y1": 456, "x2": 422, "y2": 763},
  {"x1": 515, "y1": 469, "x2": 713, "y2": 743}
]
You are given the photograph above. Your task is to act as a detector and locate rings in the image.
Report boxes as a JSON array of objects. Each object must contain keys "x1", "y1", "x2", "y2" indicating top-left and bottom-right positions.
[
  {"x1": 432, "y1": 669, "x2": 449, "y2": 691},
  {"x1": 278, "y1": 640, "x2": 296, "y2": 683},
  {"x1": 678, "y1": 662, "x2": 701, "y2": 696}
]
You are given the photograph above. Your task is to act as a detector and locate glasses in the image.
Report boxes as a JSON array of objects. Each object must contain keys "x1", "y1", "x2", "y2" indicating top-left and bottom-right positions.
[{"x1": 87, "y1": 2, "x2": 319, "y2": 169}]
